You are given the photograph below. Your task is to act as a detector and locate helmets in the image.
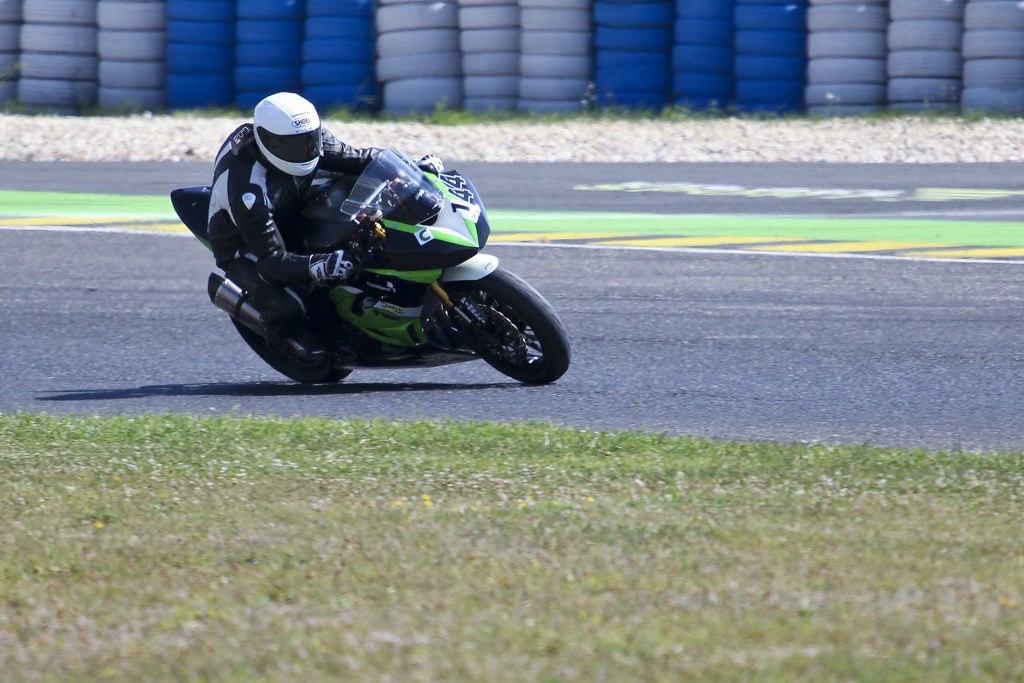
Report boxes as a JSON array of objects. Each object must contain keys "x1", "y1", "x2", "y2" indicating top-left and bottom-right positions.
[{"x1": 253, "y1": 92, "x2": 322, "y2": 177}]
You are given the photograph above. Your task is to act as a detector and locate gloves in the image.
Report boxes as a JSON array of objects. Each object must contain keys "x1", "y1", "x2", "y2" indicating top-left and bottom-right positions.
[
  {"x1": 416, "y1": 155, "x2": 444, "y2": 176},
  {"x1": 307, "y1": 247, "x2": 359, "y2": 282}
]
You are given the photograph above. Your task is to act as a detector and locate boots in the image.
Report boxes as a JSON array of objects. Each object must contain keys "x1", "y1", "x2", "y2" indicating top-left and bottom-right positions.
[{"x1": 258, "y1": 316, "x2": 326, "y2": 370}]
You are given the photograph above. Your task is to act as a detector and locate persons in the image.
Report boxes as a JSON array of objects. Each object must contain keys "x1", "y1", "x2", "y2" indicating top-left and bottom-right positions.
[{"x1": 206, "y1": 92, "x2": 444, "y2": 372}]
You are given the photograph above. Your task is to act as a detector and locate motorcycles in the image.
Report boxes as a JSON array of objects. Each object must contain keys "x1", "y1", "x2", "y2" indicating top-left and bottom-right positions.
[{"x1": 171, "y1": 152, "x2": 572, "y2": 383}]
